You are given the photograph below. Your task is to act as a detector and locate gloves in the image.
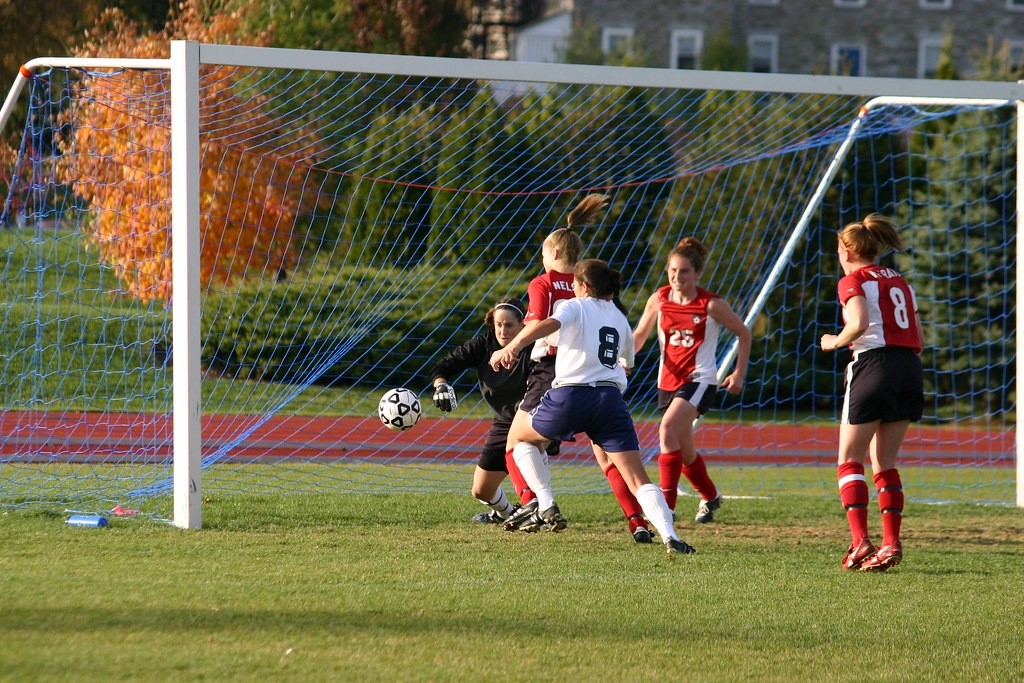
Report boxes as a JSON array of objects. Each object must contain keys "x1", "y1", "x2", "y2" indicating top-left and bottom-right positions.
[{"x1": 432, "y1": 382, "x2": 457, "y2": 412}]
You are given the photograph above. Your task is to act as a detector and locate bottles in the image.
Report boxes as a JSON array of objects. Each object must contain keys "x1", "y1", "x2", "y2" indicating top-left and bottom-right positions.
[{"x1": 64, "y1": 515, "x2": 108, "y2": 528}]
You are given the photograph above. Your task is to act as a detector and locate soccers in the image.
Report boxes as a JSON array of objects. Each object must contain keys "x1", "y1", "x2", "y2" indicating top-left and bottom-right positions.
[{"x1": 377, "y1": 387, "x2": 422, "y2": 431}]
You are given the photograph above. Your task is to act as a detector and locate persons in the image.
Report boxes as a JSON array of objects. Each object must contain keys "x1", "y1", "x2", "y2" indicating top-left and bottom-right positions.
[
  {"x1": 487, "y1": 193, "x2": 696, "y2": 553},
  {"x1": 631, "y1": 235, "x2": 752, "y2": 523},
  {"x1": 429, "y1": 299, "x2": 560, "y2": 525},
  {"x1": 821, "y1": 211, "x2": 923, "y2": 572}
]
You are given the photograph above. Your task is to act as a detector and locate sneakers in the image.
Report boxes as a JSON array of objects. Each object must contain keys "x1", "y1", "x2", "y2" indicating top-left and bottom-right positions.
[
  {"x1": 472, "y1": 511, "x2": 503, "y2": 523},
  {"x1": 842, "y1": 537, "x2": 877, "y2": 570},
  {"x1": 632, "y1": 527, "x2": 652, "y2": 543},
  {"x1": 502, "y1": 498, "x2": 539, "y2": 532},
  {"x1": 668, "y1": 540, "x2": 696, "y2": 554},
  {"x1": 695, "y1": 490, "x2": 724, "y2": 524},
  {"x1": 858, "y1": 541, "x2": 902, "y2": 571},
  {"x1": 520, "y1": 501, "x2": 567, "y2": 533}
]
